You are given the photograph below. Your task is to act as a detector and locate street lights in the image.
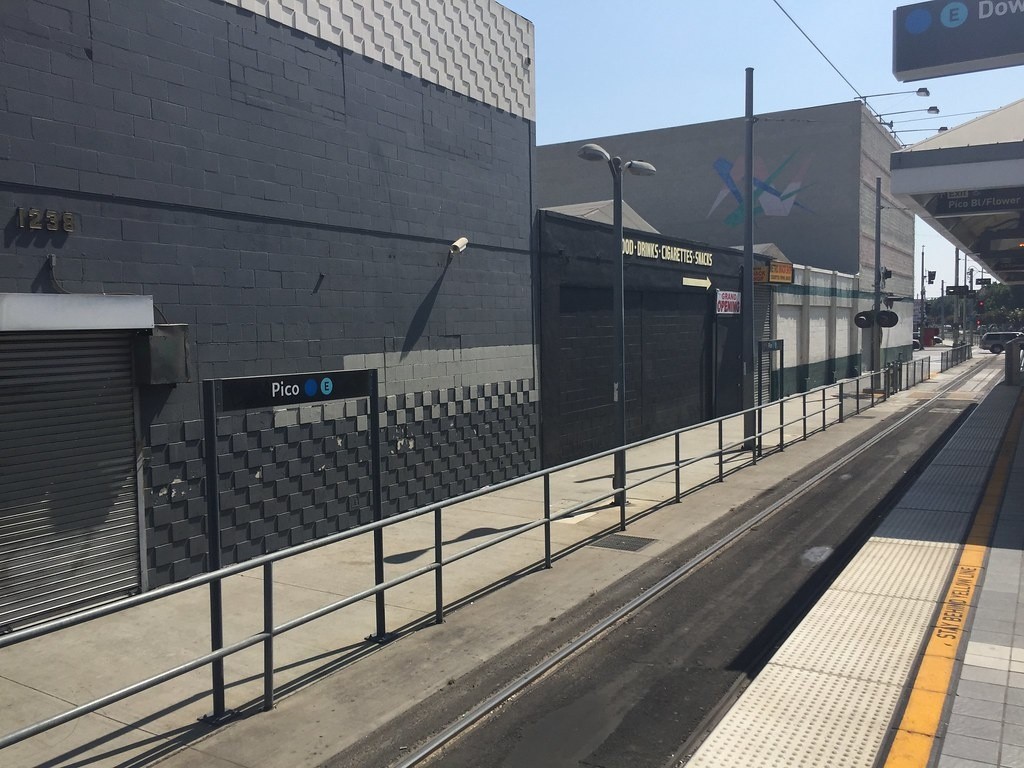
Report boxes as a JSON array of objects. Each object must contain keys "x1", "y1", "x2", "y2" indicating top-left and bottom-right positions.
[{"x1": 578, "y1": 142, "x2": 656, "y2": 504}]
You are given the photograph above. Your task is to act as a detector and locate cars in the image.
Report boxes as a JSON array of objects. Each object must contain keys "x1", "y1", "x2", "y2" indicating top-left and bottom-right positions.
[
  {"x1": 977, "y1": 325, "x2": 987, "y2": 334},
  {"x1": 988, "y1": 324, "x2": 997, "y2": 332},
  {"x1": 934, "y1": 338, "x2": 942, "y2": 343},
  {"x1": 913, "y1": 340, "x2": 919, "y2": 349}
]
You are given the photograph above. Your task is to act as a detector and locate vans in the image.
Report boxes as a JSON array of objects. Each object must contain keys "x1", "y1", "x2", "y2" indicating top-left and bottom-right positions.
[{"x1": 979, "y1": 332, "x2": 1024, "y2": 354}]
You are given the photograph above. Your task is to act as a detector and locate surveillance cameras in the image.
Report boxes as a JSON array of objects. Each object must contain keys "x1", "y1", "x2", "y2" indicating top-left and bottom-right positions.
[{"x1": 451, "y1": 237, "x2": 468, "y2": 254}]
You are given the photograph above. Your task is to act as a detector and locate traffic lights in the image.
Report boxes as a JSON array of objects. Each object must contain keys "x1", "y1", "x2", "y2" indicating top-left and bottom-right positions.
[
  {"x1": 926, "y1": 303, "x2": 931, "y2": 314},
  {"x1": 979, "y1": 301, "x2": 983, "y2": 308},
  {"x1": 929, "y1": 271, "x2": 936, "y2": 284}
]
